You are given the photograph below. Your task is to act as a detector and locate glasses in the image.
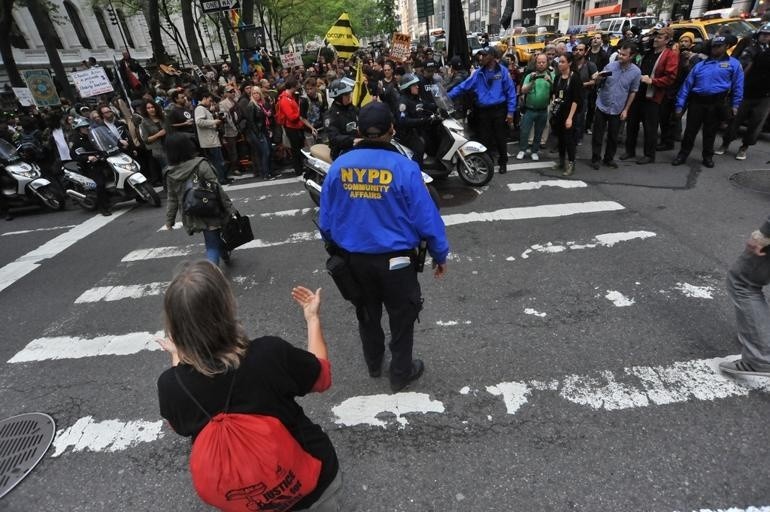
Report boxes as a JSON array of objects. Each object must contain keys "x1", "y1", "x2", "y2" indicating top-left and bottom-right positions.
[
  {"x1": 102, "y1": 110, "x2": 113, "y2": 115},
  {"x1": 574, "y1": 48, "x2": 584, "y2": 52},
  {"x1": 226, "y1": 90, "x2": 236, "y2": 94}
]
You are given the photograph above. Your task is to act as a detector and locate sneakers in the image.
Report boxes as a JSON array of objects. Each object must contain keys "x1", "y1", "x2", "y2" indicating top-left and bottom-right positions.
[
  {"x1": 516, "y1": 150, "x2": 525, "y2": 160},
  {"x1": 587, "y1": 128, "x2": 592, "y2": 135},
  {"x1": 218, "y1": 166, "x2": 304, "y2": 187},
  {"x1": 714, "y1": 143, "x2": 729, "y2": 155},
  {"x1": 718, "y1": 357, "x2": 769, "y2": 377},
  {"x1": 530, "y1": 152, "x2": 539, "y2": 161},
  {"x1": 735, "y1": 145, "x2": 747, "y2": 160}
]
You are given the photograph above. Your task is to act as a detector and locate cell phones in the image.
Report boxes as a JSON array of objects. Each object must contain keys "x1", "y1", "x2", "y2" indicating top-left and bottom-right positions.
[{"x1": 602, "y1": 72, "x2": 613, "y2": 77}]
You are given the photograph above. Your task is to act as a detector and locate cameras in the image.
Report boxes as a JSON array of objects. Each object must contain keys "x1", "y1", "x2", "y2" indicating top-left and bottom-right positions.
[{"x1": 536, "y1": 75, "x2": 544, "y2": 78}]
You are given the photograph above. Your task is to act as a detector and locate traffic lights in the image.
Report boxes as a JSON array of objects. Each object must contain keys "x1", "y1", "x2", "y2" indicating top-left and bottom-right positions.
[{"x1": 240, "y1": 26, "x2": 267, "y2": 50}]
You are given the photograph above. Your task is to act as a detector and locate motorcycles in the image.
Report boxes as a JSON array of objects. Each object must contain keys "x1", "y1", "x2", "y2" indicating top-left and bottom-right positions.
[
  {"x1": 0, "y1": 135, "x2": 68, "y2": 213},
  {"x1": 56, "y1": 123, "x2": 164, "y2": 213},
  {"x1": 299, "y1": 130, "x2": 443, "y2": 215},
  {"x1": 387, "y1": 82, "x2": 497, "y2": 188}
]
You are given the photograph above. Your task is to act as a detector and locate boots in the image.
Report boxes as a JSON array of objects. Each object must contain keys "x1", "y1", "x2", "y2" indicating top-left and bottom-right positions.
[{"x1": 561, "y1": 159, "x2": 577, "y2": 176}]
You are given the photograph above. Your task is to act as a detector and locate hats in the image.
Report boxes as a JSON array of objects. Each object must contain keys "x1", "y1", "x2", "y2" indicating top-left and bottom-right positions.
[
  {"x1": 357, "y1": 100, "x2": 394, "y2": 138},
  {"x1": 476, "y1": 45, "x2": 499, "y2": 59},
  {"x1": 710, "y1": 34, "x2": 727, "y2": 47},
  {"x1": 79, "y1": 106, "x2": 89, "y2": 111},
  {"x1": 678, "y1": 31, "x2": 695, "y2": 45},
  {"x1": 447, "y1": 55, "x2": 464, "y2": 66},
  {"x1": 655, "y1": 26, "x2": 674, "y2": 38},
  {"x1": 166, "y1": 87, "x2": 178, "y2": 98},
  {"x1": 224, "y1": 85, "x2": 234, "y2": 92}
]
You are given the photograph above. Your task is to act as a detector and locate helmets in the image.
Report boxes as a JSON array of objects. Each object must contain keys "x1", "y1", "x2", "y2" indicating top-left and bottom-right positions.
[
  {"x1": 71, "y1": 116, "x2": 91, "y2": 130},
  {"x1": 756, "y1": 22, "x2": 770, "y2": 35},
  {"x1": 328, "y1": 76, "x2": 355, "y2": 98},
  {"x1": 398, "y1": 72, "x2": 421, "y2": 92}
]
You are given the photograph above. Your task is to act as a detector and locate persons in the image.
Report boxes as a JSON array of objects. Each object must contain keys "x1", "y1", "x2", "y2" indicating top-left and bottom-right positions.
[
  {"x1": 151, "y1": 258, "x2": 349, "y2": 512},
  {"x1": 316, "y1": 101, "x2": 450, "y2": 393},
  {"x1": 710, "y1": 216, "x2": 770, "y2": 376},
  {"x1": 162, "y1": 130, "x2": 240, "y2": 271},
  {"x1": 2, "y1": 13, "x2": 769, "y2": 221}
]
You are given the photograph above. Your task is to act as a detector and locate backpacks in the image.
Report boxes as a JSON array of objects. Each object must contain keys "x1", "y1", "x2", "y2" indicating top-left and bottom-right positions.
[
  {"x1": 172, "y1": 361, "x2": 325, "y2": 512},
  {"x1": 274, "y1": 93, "x2": 288, "y2": 125}
]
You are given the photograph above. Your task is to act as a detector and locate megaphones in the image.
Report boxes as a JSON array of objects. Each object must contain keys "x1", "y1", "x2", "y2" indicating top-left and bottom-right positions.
[{"x1": 89, "y1": 56, "x2": 97, "y2": 65}]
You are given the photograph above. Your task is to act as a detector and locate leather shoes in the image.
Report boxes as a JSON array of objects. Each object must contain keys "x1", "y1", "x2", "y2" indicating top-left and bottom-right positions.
[
  {"x1": 619, "y1": 152, "x2": 636, "y2": 160},
  {"x1": 702, "y1": 157, "x2": 714, "y2": 168},
  {"x1": 590, "y1": 162, "x2": 600, "y2": 170},
  {"x1": 656, "y1": 141, "x2": 674, "y2": 151},
  {"x1": 603, "y1": 157, "x2": 618, "y2": 169},
  {"x1": 672, "y1": 152, "x2": 687, "y2": 166},
  {"x1": 499, "y1": 163, "x2": 507, "y2": 173},
  {"x1": 389, "y1": 360, "x2": 424, "y2": 393},
  {"x1": 368, "y1": 365, "x2": 385, "y2": 378},
  {"x1": 636, "y1": 154, "x2": 655, "y2": 164}
]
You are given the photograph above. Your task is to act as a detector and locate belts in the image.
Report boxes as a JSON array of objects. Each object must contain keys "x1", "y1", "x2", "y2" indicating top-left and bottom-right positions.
[{"x1": 526, "y1": 106, "x2": 547, "y2": 112}]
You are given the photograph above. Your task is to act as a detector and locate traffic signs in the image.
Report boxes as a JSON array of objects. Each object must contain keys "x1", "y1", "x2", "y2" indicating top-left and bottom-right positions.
[{"x1": 199, "y1": 0, "x2": 241, "y2": 13}]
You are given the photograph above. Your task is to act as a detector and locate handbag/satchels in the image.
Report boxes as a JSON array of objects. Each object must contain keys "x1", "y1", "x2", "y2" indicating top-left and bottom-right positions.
[
  {"x1": 181, "y1": 158, "x2": 226, "y2": 218},
  {"x1": 219, "y1": 209, "x2": 255, "y2": 251},
  {"x1": 326, "y1": 255, "x2": 362, "y2": 301}
]
